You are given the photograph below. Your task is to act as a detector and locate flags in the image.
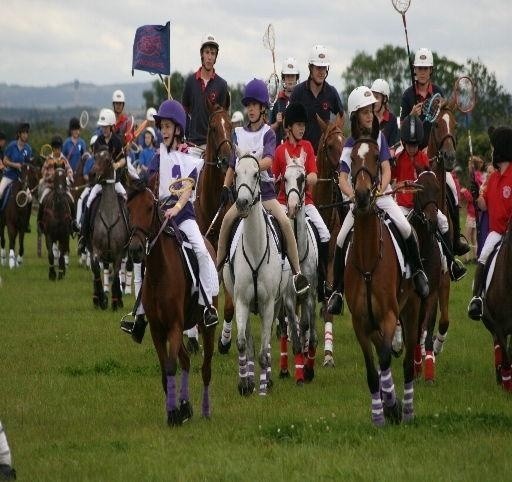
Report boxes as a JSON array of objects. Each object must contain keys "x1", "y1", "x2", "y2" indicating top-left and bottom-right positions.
[{"x1": 132, "y1": 22, "x2": 171, "y2": 76}]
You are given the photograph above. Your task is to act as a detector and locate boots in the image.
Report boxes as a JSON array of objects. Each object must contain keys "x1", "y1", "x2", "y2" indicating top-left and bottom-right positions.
[
  {"x1": 469, "y1": 262, "x2": 485, "y2": 321},
  {"x1": 404, "y1": 231, "x2": 429, "y2": 298},
  {"x1": 78, "y1": 205, "x2": 90, "y2": 249},
  {"x1": 328, "y1": 243, "x2": 344, "y2": 314},
  {"x1": 452, "y1": 204, "x2": 471, "y2": 256},
  {"x1": 318, "y1": 242, "x2": 328, "y2": 302},
  {"x1": 436, "y1": 230, "x2": 464, "y2": 281}
]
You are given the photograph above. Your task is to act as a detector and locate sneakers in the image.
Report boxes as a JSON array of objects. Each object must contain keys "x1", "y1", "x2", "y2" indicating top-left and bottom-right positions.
[
  {"x1": 201, "y1": 305, "x2": 218, "y2": 324},
  {"x1": 121, "y1": 321, "x2": 146, "y2": 343}
]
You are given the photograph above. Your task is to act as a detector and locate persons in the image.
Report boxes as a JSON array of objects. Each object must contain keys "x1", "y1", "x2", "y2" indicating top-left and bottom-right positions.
[{"x1": 0, "y1": 419, "x2": 13, "y2": 482}]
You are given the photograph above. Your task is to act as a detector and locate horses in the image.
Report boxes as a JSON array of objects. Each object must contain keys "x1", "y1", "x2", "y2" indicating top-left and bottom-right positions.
[
  {"x1": 222, "y1": 144, "x2": 320, "y2": 398},
  {"x1": 404, "y1": 93, "x2": 461, "y2": 388},
  {"x1": 191, "y1": 95, "x2": 349, "y2": 367},
  {"x1": 0, "y1": 148, "x2": 94, "y2": 282},
  {"x1": 119, "y1": 169, "x2": 219, "y2": 428},
  {"x1": 341, "y1": 111, "x2": 414, "y2": 428},
  {"x1": 91, "y1": 134, "x2": 147, "y2": 312},
  {"x1": 481, "y1": 215, "x2": 512, "y2": 391}
]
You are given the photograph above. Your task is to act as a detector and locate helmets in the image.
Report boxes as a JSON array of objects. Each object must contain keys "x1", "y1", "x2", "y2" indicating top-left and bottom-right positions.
[
  {"x1": 348, "y1": 79, "x2": 390, "y2": 120},
  {"x1": 112, "y1": 91, "x2": 124, "y2": 107},
  {"x1": 97, "y1": 109, "x2": 117, "y2": 127},
  {"x1": 285, "y1": 103, "x2": 308, "y2": 129},
  {"x1": 241, "y1": 78, "x2": 269, "y2": 106},
  {"x1": 488, "y1": 126, "x2": 512, "y2": 163},
  {"x1": 51, "y1": 136, "x2": 62, "y2": 146},
  {"x1": 146, "y1": 100, "x2": 186, "y2": 139},
  {"x1": 231, "y1": 111, "x2": 244, "y2": 123},
  {"x1": 401, "y1": 116, "x2": 424, "y2": 142},
  {"x1": 200, "y1": 34, "x2": 219, "y2": 51},
  {"x1": 414, "y1": 48, "x2": 433, "y2": 67},
  {"x1": 308, "y1": 45, "x2": 330, "y2": 67},
  {"x1": 16, "y1": 123, "x2": 29, "y2": 133},
  {"x1": 70, "y1": 118, "x2": 80, "y2": 129},
  {"x1": 282, "y1": 57, "x2": 299, "y2": 80}
]
